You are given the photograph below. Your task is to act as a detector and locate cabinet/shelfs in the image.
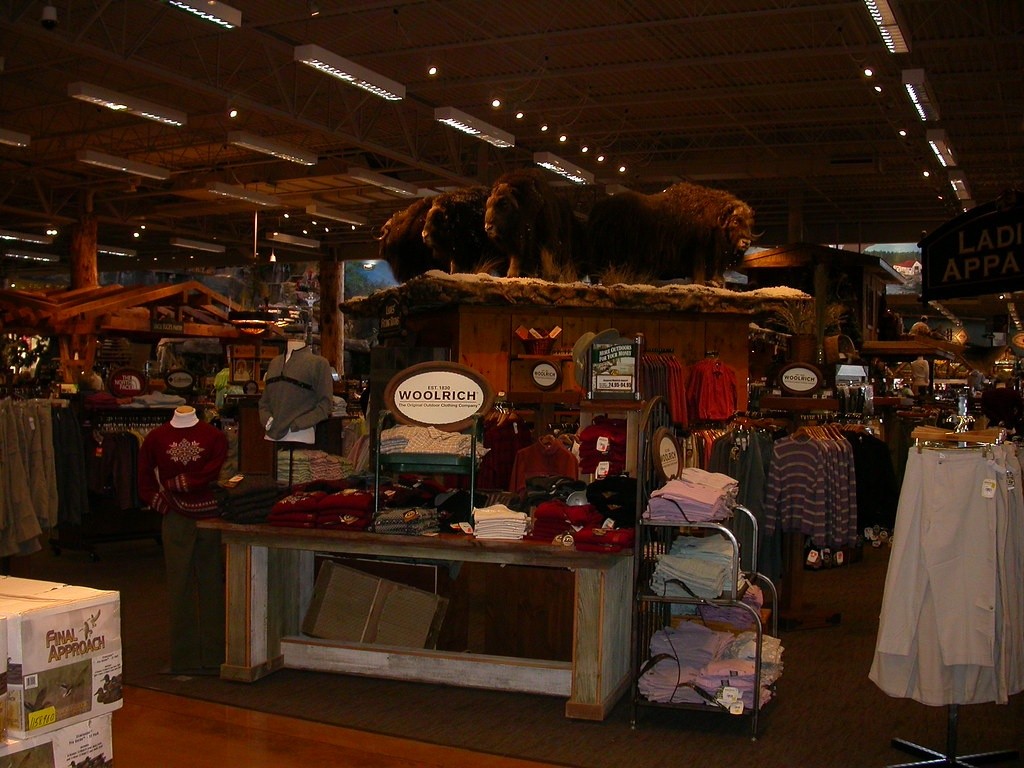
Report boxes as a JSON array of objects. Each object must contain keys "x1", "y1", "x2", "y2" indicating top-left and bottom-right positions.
[{"x1": 629, "y1": 396, "x2": 779, "y2": 741}]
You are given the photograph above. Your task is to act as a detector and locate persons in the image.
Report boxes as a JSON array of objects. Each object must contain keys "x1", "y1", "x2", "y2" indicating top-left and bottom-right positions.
[
  {"x1": 137, "y1": 405, "x2": 229, "y2": 675},
  {"x1": 259, "y1": 337, "x2": 334, "y2": 445},
  {"x1": 985, "y1": 383, "x2": 1024, "y2": 441},
  {"x1": 911, "y1": 355, "x2": 930, "y2": 386},
  {"x1": 837, "y1": 307, "x2": 948, "y2": 341}
]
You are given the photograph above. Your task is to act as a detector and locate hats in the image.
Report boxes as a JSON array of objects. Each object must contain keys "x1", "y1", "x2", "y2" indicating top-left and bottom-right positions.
[{"x1": 572, "y1": 328, "x2": 619, "y2": 367}]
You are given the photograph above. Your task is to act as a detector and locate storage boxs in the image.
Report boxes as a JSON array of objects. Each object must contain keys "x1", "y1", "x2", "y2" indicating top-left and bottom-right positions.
[
  {"x1": 0, "y1": 711, "x2": 114, "y2": 768},
  {"x1": 0, "y1": 576, "x2": 122, "y2": 741}
]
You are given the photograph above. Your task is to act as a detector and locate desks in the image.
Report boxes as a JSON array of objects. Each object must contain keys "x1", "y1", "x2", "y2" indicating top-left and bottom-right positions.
[{"x1": 194, "y1": 513, "x2": 633, "y2": 722}]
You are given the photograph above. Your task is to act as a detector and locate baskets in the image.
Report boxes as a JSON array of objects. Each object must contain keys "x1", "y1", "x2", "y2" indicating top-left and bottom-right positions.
[{"x1": 521, "y1": 328, "x2": 557, "y2": 356}]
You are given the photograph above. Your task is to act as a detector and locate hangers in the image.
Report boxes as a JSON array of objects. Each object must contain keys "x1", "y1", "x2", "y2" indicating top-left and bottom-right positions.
[
  {"x1": 0, "y1": 383, "x2": 72, "y2": 408},
  {"x1": 84, "y1": 405, "x2": 204, "y2": 434},
  {"x1": 483, "y1": 347, "x2": 1008, "y2": 453}
]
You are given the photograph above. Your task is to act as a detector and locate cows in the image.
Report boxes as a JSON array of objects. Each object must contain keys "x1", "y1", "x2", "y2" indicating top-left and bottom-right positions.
[{"x1": 369, "y1": 170, "x2": 764, "y2": 289}]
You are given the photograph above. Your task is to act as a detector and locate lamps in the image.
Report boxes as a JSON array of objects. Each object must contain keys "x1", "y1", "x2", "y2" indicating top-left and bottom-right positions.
[
  {"x1": 97, "y1": 245, "x2": 136, "y2": 257},
  {"x1": 534, "y1": 152, "x2": 594, "y2": 185},
  {"x1": 948, "y1": 170, "x2": 972, "y2": 200},
  {"x1": 348, "y1": 167, "x2": 418, "y2": 196},
  {"x1": 926, "y1": 129, "x2": 958, "y2": 167},
  {"x1": 206, "y1": 181, "x2": 275, "y2": 207},
  {"x1": 434, "y1": 106, "x2": 515, "y2": 148},
  {"x1": 66, "y1": 81, "x2": 187, "y2": 128},
  {"x1": 168, "y1": 0, "x2": 242, "y2": 29},
  {"x1": 901, "y1": 69, "x2": 941, "y2": 122},
  {"x1": 928, "y1": 293, "x2": 1022, "y2": 331},
  {"x1": 169, "y1": 238, "x2": 225, "y2": 253},
  {"x1": 293, "y1": 45, "x2": 406, "y2": 101},
  {"x1": 862, "y1": 0, "x2": 911, "y2": 54},
  {"x1": 76, "y1": 148, "x2": 170, "y2": 180},
  {"x1": 266, "y1": 232, "x2": 320, "y2": 249},
  {"x1": 0, "y1": 229, "x2": 59, "y2": 262},
  {"x1": 228, "y1": 131, "x2": 318, "y2": 165},
  {"x1": 0, "y1": 128, "x2": 30, "y2": 147},
  {"x1": 305, "y1": 204, "x2": 367, "y2": 226}
]
